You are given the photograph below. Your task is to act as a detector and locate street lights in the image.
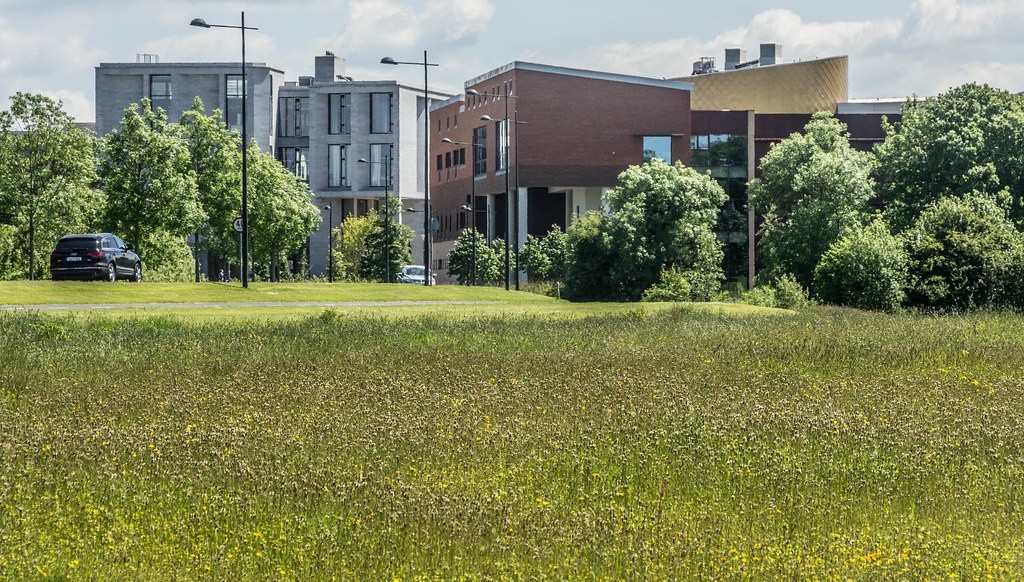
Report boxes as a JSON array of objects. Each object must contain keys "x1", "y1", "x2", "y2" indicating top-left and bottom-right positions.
[
  {"x1": 379, "y1": 49, "x2": 441, "y2": 286},
  {"x1": 479, "y1": 109, "x2": 528, "y2": 291},
  {"x1": 357, "y1": 154, "x2": 399, "y2": 283},
  {"x1": 442, "y1": 128, "x2": 486, "y2": 286},
  {"x1": 460, "y1": 202, "x2": 498, "y2": 285},
  {"x1": 405, "y1": 204, "x2": 440, "y2": 286},
  {"x1": 325, "y1": 202, "x2": 333, "y2": 282},
  {"x1": 464, "y1": 81, "x2": 519, "y2": 291},
  {"x1": 187, "y1": 11, "x2": 259, "y2": 289}
]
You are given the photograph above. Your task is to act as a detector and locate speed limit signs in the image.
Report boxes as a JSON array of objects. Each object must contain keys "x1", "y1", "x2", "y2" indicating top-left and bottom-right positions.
[{"x1": 233, "y1": 217, "x2": 243, "y2": 233}]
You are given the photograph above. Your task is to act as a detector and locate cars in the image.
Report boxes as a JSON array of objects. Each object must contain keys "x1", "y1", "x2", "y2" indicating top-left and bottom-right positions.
[{"x1": 395, "y1": 265, "x2": 437, "y2": 286}]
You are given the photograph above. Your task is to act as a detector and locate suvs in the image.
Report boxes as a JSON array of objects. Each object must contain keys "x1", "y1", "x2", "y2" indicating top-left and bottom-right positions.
[{"x1": 48, "y1": 232, "x2": 143, "y2": 283}]
